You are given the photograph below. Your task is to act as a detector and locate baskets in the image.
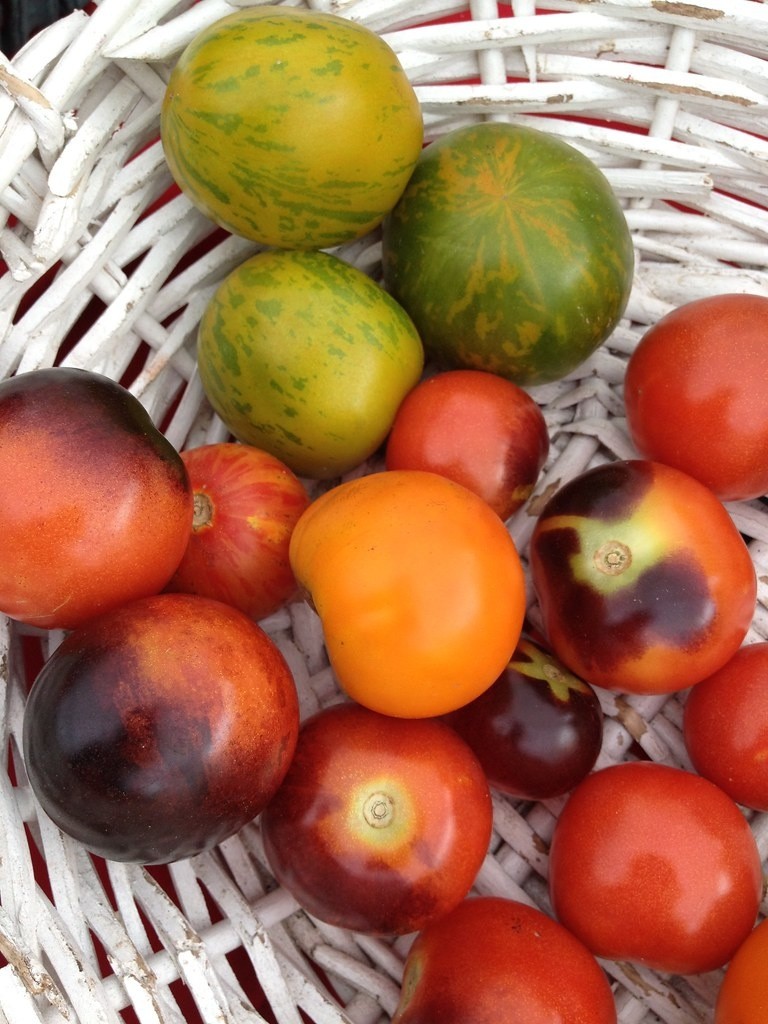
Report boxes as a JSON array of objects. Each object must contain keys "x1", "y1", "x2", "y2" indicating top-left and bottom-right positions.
[{"x1": 0, "y1": 0, "x2": 768, "y2": 1024}]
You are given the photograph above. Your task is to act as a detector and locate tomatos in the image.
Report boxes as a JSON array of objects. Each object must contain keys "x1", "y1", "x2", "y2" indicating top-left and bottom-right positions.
[{"x1": 0, "y1": 7, "x2": 768, "y2": 1024}]
